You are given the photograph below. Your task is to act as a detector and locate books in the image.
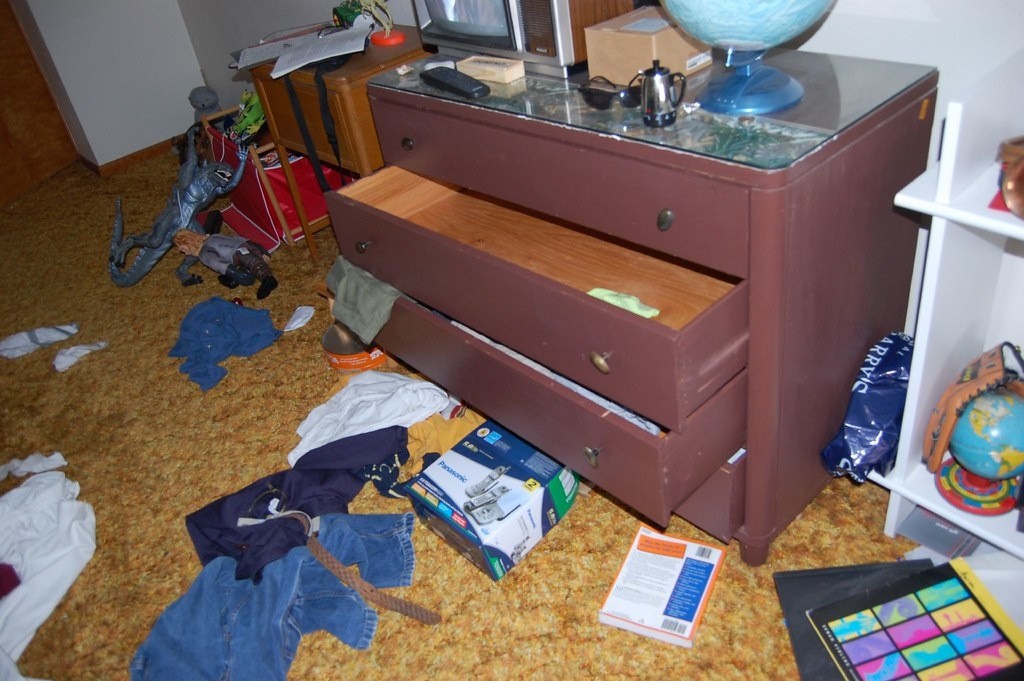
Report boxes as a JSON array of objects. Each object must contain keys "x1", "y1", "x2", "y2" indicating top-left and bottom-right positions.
[
  {"x1": 598, "y1": 521, "x2": 727, "y2": 651},
  {"x1": 772, "y1": 505, "x2": 1024, "y2": 681}
]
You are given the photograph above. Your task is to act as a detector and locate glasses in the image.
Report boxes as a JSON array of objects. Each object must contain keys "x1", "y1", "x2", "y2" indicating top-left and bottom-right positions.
[{"x1": 577, "y1": 71, "x2": 643, "y2": 110}]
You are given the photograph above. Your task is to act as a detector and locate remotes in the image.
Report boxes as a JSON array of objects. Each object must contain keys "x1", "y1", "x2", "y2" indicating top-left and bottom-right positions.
[{"x1": 419, "y1": 66, "x2": 490, "y2": 99}]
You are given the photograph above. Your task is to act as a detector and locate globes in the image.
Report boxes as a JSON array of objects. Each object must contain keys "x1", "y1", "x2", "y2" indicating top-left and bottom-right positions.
[
  {"x1": 935, "y1": 387, "x2": 1024, "y2": 517},
  {"x1": 659, "y1": 0, "x2": 838, "y2": 117}
]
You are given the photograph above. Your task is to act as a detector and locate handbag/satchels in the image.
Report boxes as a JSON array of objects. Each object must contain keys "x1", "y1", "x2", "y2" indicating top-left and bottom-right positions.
[
  {"x1": 819, "y1": 330, "x2": 915, "y2": 486},
  {"x1": 297, "y1": 35, "x2": 370, "y2": 69}
]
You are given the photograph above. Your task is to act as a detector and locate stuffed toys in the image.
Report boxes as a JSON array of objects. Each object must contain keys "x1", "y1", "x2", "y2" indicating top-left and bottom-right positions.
[{"x1": 187, "y1": 86, "x2": 223, "y2": 122}]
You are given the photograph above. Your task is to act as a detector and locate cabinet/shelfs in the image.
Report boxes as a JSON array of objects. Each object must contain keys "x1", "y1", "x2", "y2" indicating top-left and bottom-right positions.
[
  {"x1": 322, "y1": 46, "x2": 940, "y2": 567},
  {"x1": 867, "y1": 99, "x2": 1024, "y2": 565}
]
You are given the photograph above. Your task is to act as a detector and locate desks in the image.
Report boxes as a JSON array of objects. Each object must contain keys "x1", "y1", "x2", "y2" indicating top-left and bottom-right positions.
[{"x1": 248, "y1": 23, "x2": 438, "y2": 263}]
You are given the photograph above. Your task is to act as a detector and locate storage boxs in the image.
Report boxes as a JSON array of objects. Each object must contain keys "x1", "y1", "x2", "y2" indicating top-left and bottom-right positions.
[
  {"x1": 404, "y1": 420, "x2": 580, "y2": 579},
  {"x1": 201, "y1": 105, "x2": 358, "y2": 254}
]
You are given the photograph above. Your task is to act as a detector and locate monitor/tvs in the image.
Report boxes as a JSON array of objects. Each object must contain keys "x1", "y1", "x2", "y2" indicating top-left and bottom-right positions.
[{"x1": 411, "y1": 0, "x2": 634, "y2": 79}]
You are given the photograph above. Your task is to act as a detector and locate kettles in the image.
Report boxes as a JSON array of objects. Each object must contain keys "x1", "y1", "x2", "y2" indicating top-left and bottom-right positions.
[{"x1": 638, "y1": 60, "x2": 685, "y2": 127}]
